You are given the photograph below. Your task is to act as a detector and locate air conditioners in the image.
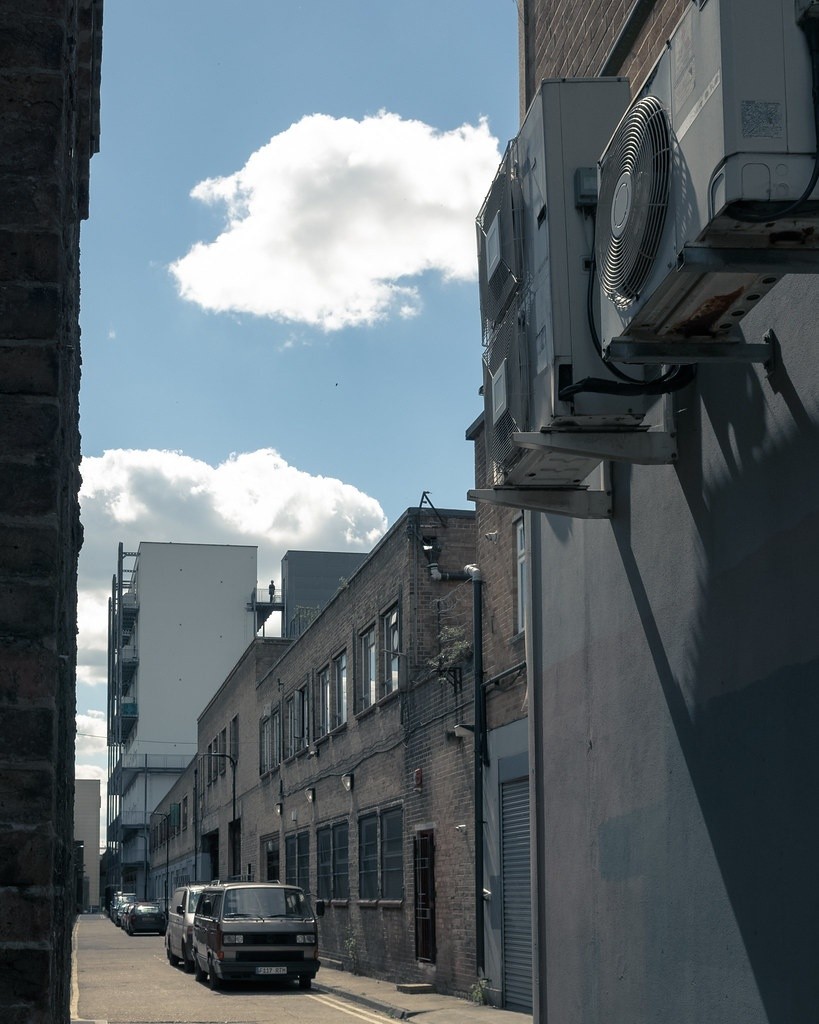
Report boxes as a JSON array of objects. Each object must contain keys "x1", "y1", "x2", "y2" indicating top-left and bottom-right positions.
[
  {"x1": 474, "y1": 76, "x2": 644, "y2": 491},
  {"x1": 596, "y1": 1, "x2": 819, "y2": 362}
]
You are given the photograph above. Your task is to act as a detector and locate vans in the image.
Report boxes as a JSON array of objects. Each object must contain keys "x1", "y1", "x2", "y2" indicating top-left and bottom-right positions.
[
  {"x1": 165, "y1": 883, "x2": 224, "y2": 972},
  {"x1": 193, "y1": 878, "x2": 324, "y2": 993}
]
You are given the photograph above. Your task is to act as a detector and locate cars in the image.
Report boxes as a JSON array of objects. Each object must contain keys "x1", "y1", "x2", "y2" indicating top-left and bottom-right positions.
[{"x1": 110, "y1": 892, "x2": 167, "y2": 936}]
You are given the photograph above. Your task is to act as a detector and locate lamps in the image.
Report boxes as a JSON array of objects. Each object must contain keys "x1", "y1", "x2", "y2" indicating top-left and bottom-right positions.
[
  {"x1": 274, "y1": 802, "x2": 282, "y2": 816},
  {"x1": 341, "y1": 773, "x2": 354, "y2": 791},
  {"x1": 305, "y1": 788, "x2": 315, "y2": 803}
]
